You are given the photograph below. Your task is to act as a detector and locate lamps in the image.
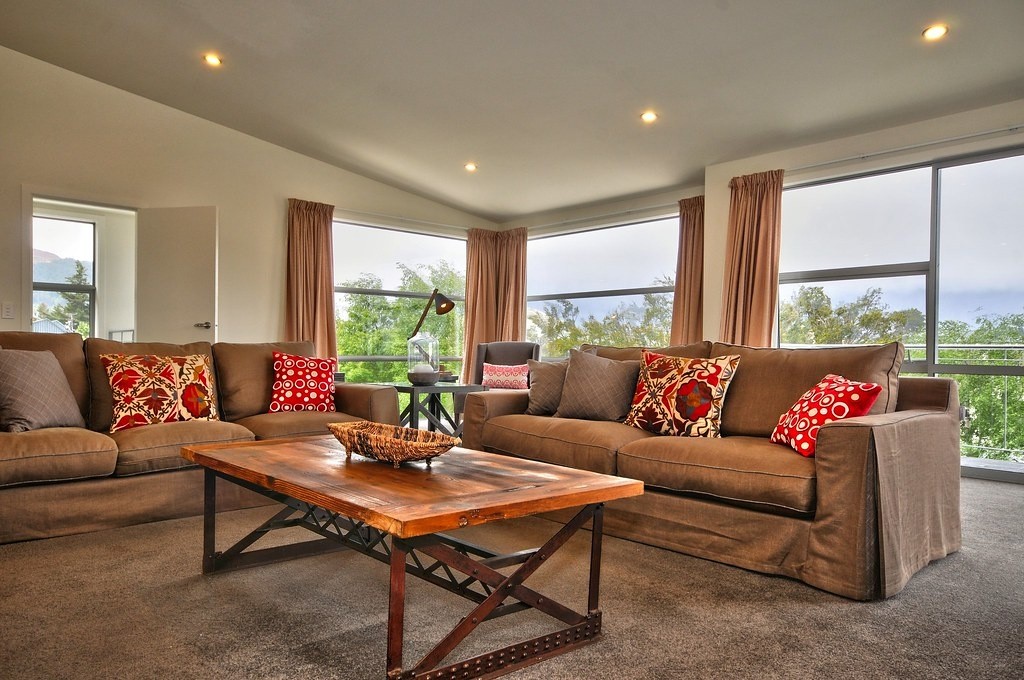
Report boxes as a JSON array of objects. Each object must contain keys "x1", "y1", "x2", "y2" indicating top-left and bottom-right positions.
[{"x1": 406, "y1": 288, "x2": 459, "y2": 381}]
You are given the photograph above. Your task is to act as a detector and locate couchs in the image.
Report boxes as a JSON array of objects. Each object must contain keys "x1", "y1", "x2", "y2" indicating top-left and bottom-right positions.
[
  {"x1": 0, "y1": 330, "x2": 400, "y2": 545},
  {"x1": 461, "y1": 341, "x2": 961, "y2": 601}
]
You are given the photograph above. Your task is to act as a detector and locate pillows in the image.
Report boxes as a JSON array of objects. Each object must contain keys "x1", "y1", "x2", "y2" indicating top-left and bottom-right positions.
[
  {"x1": 0, "y1": 349, "x2": 88, "y2": 433},
  {"x1": 100, "y1": 354, "x2": 221, "y2": 434},
  {"x1": 553, "y1": 350, "x2": 640, "y2": 421},
  {"x1": 526, "y1": 358, "x2": 568, "y2": 415},
  {"x1": 622, "y1": 350, "x2": 742, "y2": 439},
  {"x1": 482, "y1": 362, "x2": 528, "y2": 388},
  {"x1": 270, "y1": 352, "x2": 337, "y2": 415},
  {"x1": 770, "y1": 374, "x2": 882, "y2": 458}
]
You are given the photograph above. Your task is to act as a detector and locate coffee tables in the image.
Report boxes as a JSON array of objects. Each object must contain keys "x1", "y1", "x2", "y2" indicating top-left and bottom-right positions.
[{"x1": 180, "y1": 435, "x2": 645, "y2": 680}]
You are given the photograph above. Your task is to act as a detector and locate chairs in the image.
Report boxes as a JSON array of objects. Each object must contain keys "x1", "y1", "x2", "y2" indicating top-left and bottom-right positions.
[{"x1": 452, "y1": 341, "x2": 540, "y2": 424}]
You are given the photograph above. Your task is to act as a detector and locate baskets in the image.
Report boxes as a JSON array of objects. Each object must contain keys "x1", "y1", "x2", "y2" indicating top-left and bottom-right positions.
[{"x1": 326, "y1": 419, "x2": 462, "y2": 468}]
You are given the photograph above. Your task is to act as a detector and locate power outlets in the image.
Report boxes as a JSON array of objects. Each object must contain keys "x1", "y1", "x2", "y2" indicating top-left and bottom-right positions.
[{"x1": 2, "y1": 302, "x2": 16, "y2": 319}]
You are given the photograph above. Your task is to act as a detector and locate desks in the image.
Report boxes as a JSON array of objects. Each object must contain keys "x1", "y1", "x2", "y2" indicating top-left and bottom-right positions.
[{"x1": 363, "y1": 381, "x2": 490, "y2": 442}]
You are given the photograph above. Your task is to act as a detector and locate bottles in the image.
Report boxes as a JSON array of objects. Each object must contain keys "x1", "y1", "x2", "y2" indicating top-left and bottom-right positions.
[{"x1": 407, "y1": 331, "x2": 441, "y2": 385}]
